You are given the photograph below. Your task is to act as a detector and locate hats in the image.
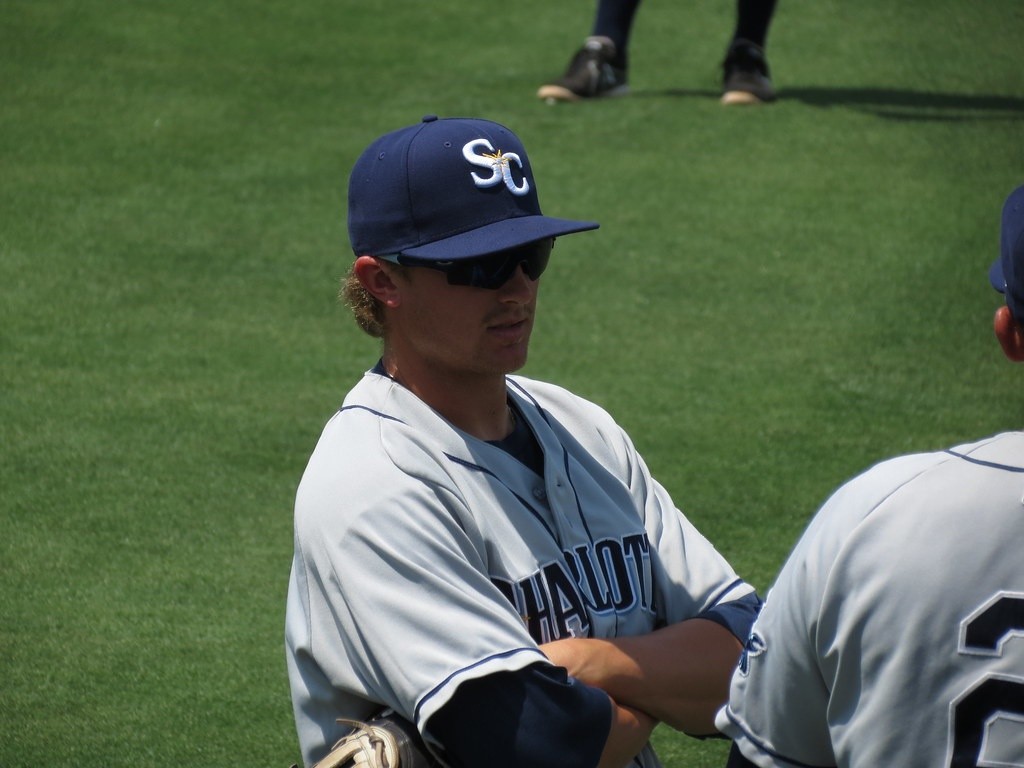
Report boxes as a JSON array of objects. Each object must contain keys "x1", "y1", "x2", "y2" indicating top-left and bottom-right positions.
[
  {"x1": 348, "y1": 115, "x2": 600, "y2": 259},
  {"x1": 990, "y1": 184, "x2": 1024, "y2": 322}
]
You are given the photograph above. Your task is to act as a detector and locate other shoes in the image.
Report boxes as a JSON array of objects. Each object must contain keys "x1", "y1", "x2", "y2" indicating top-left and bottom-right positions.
[
  {"x1": 535, "y1": 35, "x2": 631, "y2": 106},
  {"x1": 718, "y1": 38, "x2": 772, "y2": 106}
]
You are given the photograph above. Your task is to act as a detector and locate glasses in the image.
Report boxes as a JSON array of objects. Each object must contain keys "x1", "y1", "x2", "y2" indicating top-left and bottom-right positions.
[{"x1": 431, "y1": 238, "x2": 554, "y2": 290}]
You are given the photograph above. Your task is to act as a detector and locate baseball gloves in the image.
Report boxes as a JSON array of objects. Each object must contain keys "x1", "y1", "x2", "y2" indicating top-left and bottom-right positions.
[{"x1": 306, "y1": 705, "x2": 443, "y2": 768}]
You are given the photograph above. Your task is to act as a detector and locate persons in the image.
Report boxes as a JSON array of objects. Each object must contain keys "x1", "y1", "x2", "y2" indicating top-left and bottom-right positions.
[
  {"x1": 715, "y1": 187, "x2": 1024, "y2": 768},
  {"x1": 284, "y1": 113, "x2": 763, "y2": 768},
  {"x1": 537, "y1": 0, "x2": 779, "y2": 105}
]
